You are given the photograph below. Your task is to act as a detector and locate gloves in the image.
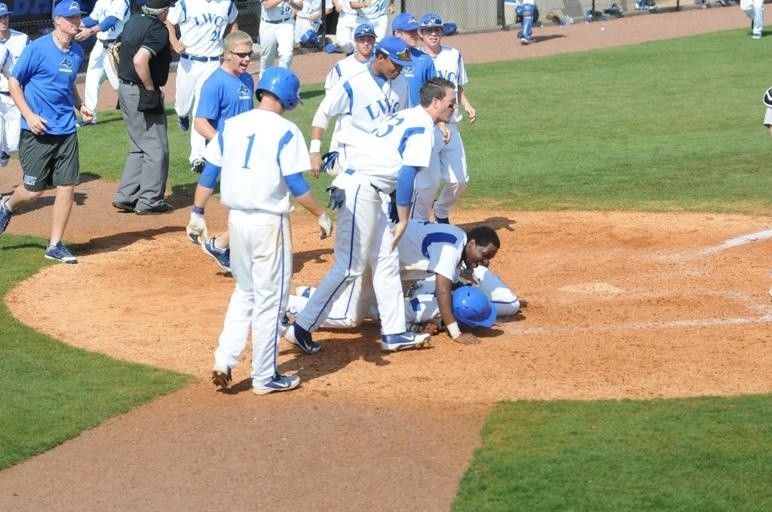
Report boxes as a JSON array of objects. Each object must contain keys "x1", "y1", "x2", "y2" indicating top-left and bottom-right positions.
[
  {"x1": 187, "y1": 213, "x2": 208, "y2": 244},
  {"x1": 318, "y1": 187, "x2": 345, "y2": 240}
]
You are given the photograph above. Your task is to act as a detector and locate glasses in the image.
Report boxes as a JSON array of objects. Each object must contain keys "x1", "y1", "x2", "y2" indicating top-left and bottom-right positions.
[{"x1": 229, "y1": 51, "x2": 252, "y2": 58}]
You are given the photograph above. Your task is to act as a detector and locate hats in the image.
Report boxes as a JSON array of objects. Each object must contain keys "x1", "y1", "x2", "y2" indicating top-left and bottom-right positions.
[
  {"x1": 53, "y1": 0, "x2": 87, "y2": 17},
  {"x1": 146, "y1": 0, "x2": 175, "y2": 8},
  {"x1": 354, "y1": 13, "x2": 443, "y2": 67},
  {"x1": 0, "y1": 2, "x2": 15, "y2": 16}
]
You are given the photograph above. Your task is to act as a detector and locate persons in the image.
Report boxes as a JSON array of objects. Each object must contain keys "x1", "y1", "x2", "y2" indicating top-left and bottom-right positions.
[
  {"x1": 0, "y1": 4, "x2": 33, "y2": 168},
  {"x1": 282, "y1": 78, "x2": 460, "y2": 356},
  {"x1": 761, "y1": 82, "x2": 772, "y2": 133},
  {"x1": 0, "y1": 2, "x2": 94, "y2": 267},
  {"x1": 74, "y1": 0, "x2": 479, "y2": 223},
  {"x1": 735, "y1": 1, "x2": 767, "y2": 42},
  {"x1": 186, "y1": 66, "x2": 337, "y2": 397},
  {"x1": 185, "y1": 31, "x2": 254, "y2": 248},
  {"x1": 510, "y1": 0, "x2": 538, "y2": 47},
  {"x1": 279, "y1": 210, "x2": 501, "y2": 347}
]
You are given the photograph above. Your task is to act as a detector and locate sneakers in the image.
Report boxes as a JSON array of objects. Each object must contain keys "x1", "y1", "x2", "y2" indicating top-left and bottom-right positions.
[
  {"x1": 191, "y1": 159, "x2": 205, "y2": 173},
  {"x1": 0, "y1": 196, "x2": 15, "y2": 234},
  {"x1": 178, "y1": 116, "x2": 189, "y2": 131},
  {"x1": 280, "y1": 287, "x2": 321, "y2": 354},
  {"x1": 45, "y1": 240, "x2": 77, "y2": 263},
  {"x1": 113, "y1": 201, "x2": 174, "y2": 214},
  {"x1": 252, "y1": 372, "x2": 300, "y2": 395},
  {"x1": 382, "y1": 331, "x2": 430, "y2": 352},
  {"x1": 202, "y1": 238, "x2": 232, "y2": 275}
]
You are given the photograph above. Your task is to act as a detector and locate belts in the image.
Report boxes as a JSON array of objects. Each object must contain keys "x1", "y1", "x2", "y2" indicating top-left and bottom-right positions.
[
  {"x1": 182, "y1": 54, "x2": 219, "y2": 62},
  {"x1": 261, "y1": 17, "x2": 290, "y2": 24},
  {"x1": 122, "y1": 79, "x2": 136, "y2": 84},
  {"x1": 99, "y1": 39, "x2": 114, "y2": 43}
]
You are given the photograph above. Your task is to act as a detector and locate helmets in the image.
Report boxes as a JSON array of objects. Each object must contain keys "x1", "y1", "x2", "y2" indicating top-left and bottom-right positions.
[
  {"x1": 256, "y1": 67, "x2": 299, "y2": 109},
  {"x1": 452, "y1": 286, "x2": 497, "y2": 328}
]
